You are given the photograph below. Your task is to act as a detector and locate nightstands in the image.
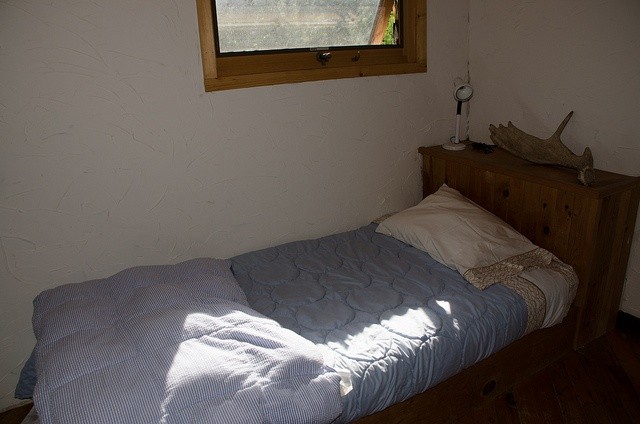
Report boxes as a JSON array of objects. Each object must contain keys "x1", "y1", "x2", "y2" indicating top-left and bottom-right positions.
[{"x1": 419, "y1": 140, "x2": 640, "y2": 350}]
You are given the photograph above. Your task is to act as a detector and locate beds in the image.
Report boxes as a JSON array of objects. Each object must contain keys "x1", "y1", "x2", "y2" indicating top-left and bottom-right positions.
[{"x1": 17, "y1": 223, "x2": 578, "y2": 423}]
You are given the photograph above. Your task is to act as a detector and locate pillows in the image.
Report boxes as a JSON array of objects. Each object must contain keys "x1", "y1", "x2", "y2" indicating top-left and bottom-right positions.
[{"x1": 375, "y1": 183, "x2": 556, "y2": 290}]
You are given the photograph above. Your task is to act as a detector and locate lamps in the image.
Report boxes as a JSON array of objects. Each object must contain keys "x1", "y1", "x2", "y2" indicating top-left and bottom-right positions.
[{"x1": 442, "y1": 77, "x2": 474, "y2": 150}]
